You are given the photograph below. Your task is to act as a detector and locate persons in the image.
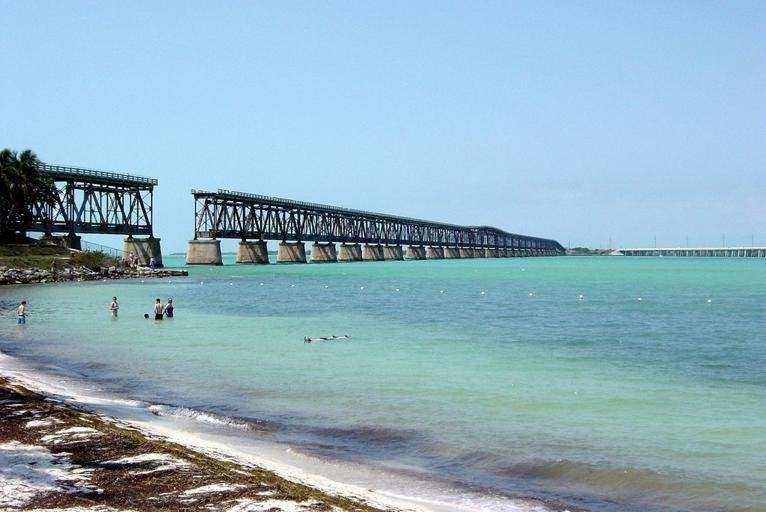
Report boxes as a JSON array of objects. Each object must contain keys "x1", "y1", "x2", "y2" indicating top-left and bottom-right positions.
[
  {"x1": 129, "y1": 252, "x2": 133, "y2": 261},
  {"x1": 109, "y1": 297, "x2": 119, "y2": 317},
  {"x1": 304, "y1": 335, "x2": 348, "y2": 342},
  {"x1": 144, "y1": 314, "x2": 149, "y2": 319},
  {"x1": 154, "y1": 299, "x2": 164, "y2": 320},
  {"x1": 164, "y1": 298, "x2": 173, "y2": 317},
  {"x1": 134, "y1": 256, "x2": 138, "y2": 265},
  {"x1": 149, "y1": 257, "x2": 156, "y2": 269},
  {"x1": 17, "y1": 301, "x2": 26, "y2": 324}
]
